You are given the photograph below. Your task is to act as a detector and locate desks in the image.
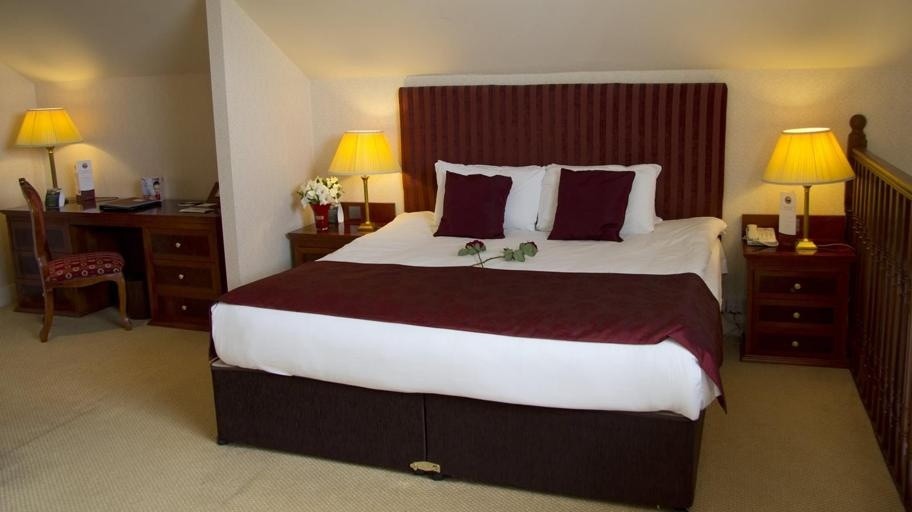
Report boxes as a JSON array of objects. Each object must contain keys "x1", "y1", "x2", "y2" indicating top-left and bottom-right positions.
[{"x1": 0, "y1": 197, "x2": 227, "y2": 330}]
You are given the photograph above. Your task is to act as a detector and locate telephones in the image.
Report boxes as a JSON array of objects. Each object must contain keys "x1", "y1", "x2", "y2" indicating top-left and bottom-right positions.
[{"x1": 746, "y1": 223, "x2": 777, "y2": 246}]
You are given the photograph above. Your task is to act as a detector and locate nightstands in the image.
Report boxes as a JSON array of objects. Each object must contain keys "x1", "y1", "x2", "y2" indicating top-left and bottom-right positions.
[
  {"x1": 741, "y1": 237, "x2": 852, "y2": 371},
  {"x1": 208, "y1": 68, "x2": 735, "y2": 511},
  {"x1": 283, "y1": 222, "x2": 378, "y2": 269}
]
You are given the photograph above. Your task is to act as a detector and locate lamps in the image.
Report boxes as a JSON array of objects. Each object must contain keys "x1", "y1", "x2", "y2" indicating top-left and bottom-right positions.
[
  {"x1": 762, "y1": 126, "x2": 857, "y2": 249},
  {"x1": 326, "y1": 130, "x2": 403, "y2": 231},
  {"x1": 13, "y1": 106, "x2": 83, "y2": 202}
]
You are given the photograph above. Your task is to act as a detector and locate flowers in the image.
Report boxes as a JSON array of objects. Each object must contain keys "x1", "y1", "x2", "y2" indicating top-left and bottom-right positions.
[
  {"x1": 297, "y1": 175, "x2": 346, "y2": 207},
  {"x1": 456, "y1": 238, "x2": 541, "y2": 274}
]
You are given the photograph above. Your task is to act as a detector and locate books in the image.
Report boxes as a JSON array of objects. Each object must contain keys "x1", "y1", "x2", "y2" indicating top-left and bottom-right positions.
[{"x1": 99, "y1": 196, "x2": 162, "y2": 212}]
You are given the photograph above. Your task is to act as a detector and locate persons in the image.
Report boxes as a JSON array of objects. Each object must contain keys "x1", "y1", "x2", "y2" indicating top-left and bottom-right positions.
[{"x1": 153, "y1": 181, "x2": 160, "y2": 200}]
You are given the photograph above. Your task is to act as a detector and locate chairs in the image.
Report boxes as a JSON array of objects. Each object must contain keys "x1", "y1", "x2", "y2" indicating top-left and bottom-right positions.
[{"x1": 13, "y1": 176, "x2": 135, "y2": 342}]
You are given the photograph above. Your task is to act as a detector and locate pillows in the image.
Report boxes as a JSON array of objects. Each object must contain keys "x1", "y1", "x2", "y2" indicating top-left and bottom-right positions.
[
  {"x1": 432, "y1": 171, "x2": 514, "y2": 241},
  {"x1": 536, "y1": 161, "x2": 663, "y2": 236},
  {"x1": 432, "y1": 159, "x2": 544, "y2": 238},
  {"x1": 548, "y1": 166, "x2": 635, "y2": 243}
]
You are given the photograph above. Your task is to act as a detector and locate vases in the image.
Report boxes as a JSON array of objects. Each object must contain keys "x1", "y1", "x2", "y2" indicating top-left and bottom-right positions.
[{"x1": 307, "y1": 202, "x2": 329, "y2": 229}]
